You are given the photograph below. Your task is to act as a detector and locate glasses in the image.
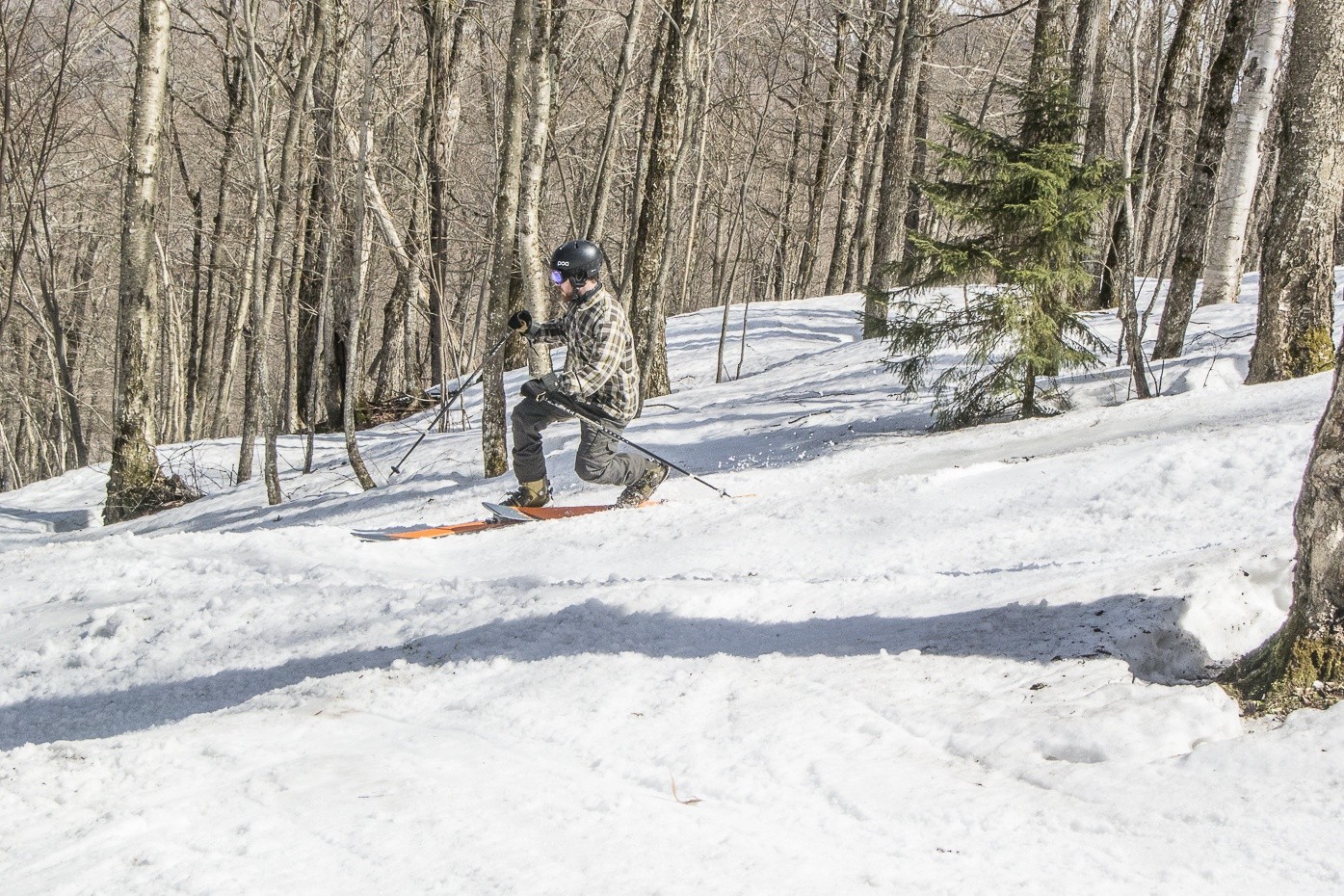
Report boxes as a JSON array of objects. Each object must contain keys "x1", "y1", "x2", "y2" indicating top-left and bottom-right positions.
[{"x1": 551, "y1": 270, "x2": 568, "y2": 284}]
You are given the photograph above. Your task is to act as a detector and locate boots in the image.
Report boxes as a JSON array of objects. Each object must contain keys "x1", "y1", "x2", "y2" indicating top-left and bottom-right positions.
[
  {"x1": 617, "y1": 459, "x2": 670, "y2": 506},
  {"x1": 493, "y1": 474, "x2": 550, "y2": 523}
]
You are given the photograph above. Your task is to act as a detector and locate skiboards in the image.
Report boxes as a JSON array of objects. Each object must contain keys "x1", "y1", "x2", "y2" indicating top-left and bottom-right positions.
[{"x1": 349, "y1": 499, "x2": 669, "y2": 541}]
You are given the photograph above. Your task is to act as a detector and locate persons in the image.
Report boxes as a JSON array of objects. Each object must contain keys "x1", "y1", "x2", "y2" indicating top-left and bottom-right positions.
[{"x1": 494, "y1": 241, "x2": 670, "y2": 519}]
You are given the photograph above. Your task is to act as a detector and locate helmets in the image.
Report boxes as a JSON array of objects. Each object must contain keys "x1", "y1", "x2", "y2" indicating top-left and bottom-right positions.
[{"x1": 550, "y1": 240, "x2": 603, "y2": 302}]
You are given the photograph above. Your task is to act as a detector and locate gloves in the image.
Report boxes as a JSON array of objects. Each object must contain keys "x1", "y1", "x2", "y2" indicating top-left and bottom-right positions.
[
  {"x1": 521, "y1": 372, "x2": 559, "y2": 403},
  {"x1": 507, "y1": 310, "x2": 541, "y2": 339}
]
